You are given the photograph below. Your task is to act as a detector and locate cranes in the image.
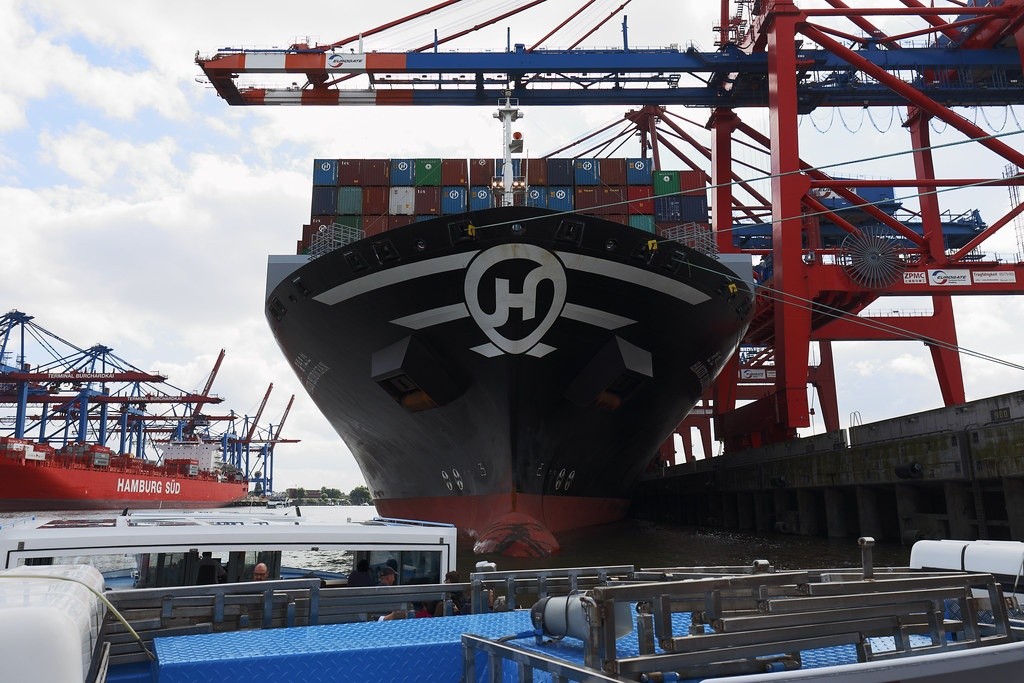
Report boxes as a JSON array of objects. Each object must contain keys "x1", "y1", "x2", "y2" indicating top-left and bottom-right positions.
[{"x1": 1, "y1": 309, "x2": 302, "y2": 508}]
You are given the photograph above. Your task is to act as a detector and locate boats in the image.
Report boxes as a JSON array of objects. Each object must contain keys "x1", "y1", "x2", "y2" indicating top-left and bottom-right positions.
[
  {"x1": 0, "y1": 459, "x2": 248, "y2": 508},
  {"x1": 267, "y1": 207, "x2": 754, "y2": 558},
  {"x1": 0, "y1": 518, "x2": 457, "y2": 595}
]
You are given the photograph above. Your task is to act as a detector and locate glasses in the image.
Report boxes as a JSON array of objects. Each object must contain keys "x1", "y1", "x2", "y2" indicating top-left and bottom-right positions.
[{"x1": 251, "y1": 573, "x2": 266, "y2": 577}]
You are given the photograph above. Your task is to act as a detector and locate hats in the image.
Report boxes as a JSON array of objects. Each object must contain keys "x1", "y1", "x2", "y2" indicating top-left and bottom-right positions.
[{"x1": 380, "y1": 566, "x2": 400, "y2": 575}]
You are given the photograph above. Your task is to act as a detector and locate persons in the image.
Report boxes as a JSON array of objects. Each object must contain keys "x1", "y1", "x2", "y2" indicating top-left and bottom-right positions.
[
  {"x1": 347, "y1": 559, "x2": 398, "y2": 586},
  {"x1": 254, "y1": 563, "x2": 267, "y2": 581},
  {"x1": 378, "y1": 601, "x2": 434, "y2": 621},
  {"x1": 202, "y1": 552, "x2": 225, "y2": 577},
  {"x1": 434, "y1": 571, "x2": 471, "y2": 617}
]
